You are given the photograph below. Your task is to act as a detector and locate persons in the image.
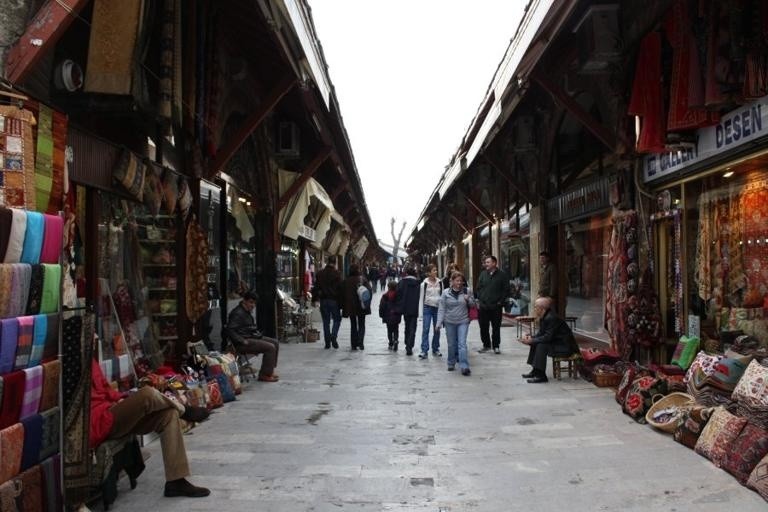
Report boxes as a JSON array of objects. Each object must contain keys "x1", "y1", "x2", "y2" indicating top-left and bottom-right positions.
[
  {"x1": 520, "y1": 296, "x2": 580, "y2": 383},
  {"x1": 226, "y1": 292, "x2": 279, "y2": 382},
  {"x1": 537, "y1": 251, "x2": 560, "y2": 314},
  {"x1": 475, "y1": 256, "x2": 510, "y2": 355},
  {"x1": 343, "y1": 264, "x2": 372, "y2": 350},
  {"x1": 310, "y1": 255, "x2": 342, "y2": 349},
  {"x1": 88, "y1": 332, "x2": 212, "y2": 497},
  {"x1": 362, "y1": 260, "x2": 477, "y2": 376}
]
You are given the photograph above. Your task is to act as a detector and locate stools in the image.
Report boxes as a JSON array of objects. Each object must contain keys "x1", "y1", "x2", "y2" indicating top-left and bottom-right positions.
[
  {"x1": 515, "y1": 315, "x2": 534, "y2": 341},
  {"x1": 563, "y1": 315, "x2": 577, "y2": 331},
  {"x1": 72, "y1": 434, "x2": 140, "y2": 512},
  {"x1": 552, "y1": 354, "x2": 583, "y2": 381}
]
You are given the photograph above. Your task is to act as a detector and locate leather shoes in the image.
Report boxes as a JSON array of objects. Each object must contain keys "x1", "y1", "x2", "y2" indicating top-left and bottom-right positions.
[
  {"x1": 522, "y1": 371, "x2": 535, "y2": 378},
  {"x1": 527, "y1": 375, "x2": 548, "y2": 383}
]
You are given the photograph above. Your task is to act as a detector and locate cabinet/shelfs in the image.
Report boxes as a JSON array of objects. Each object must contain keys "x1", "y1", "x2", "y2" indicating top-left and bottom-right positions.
[{"x1": 130, "y1": 217, "x2": 209, "y2": 354}]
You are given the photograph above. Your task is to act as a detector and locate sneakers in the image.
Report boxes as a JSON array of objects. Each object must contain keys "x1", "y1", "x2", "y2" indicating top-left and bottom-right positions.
[
  {"x1": 164, "y1": 480, "x2": 210, "y2": 497},
  {"x1": 179, "y1": 404, "x2": 209, "y2": 423}
]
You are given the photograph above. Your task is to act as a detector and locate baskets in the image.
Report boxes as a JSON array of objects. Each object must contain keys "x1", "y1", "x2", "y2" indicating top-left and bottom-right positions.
[
  {"x1": 591, "y1": 371, "x2": 623, "y2": 388},
  {"x1": 646, "y1": 392, "x2": 695, "y2": 433}
]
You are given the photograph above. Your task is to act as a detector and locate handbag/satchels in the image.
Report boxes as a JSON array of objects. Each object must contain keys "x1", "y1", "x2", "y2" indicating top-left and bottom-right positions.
[
  {"x1": 469, "y1": 304, "x2": 478, "y2": 321},
  {"x1": 357, "y1": 285, "x2": 370, "y2": 302}
]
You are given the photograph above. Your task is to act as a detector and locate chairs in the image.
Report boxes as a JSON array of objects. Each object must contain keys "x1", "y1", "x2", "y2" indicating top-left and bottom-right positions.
[{"x1": 217, "y1": 329, "x2": 257, "y2": 384}]
